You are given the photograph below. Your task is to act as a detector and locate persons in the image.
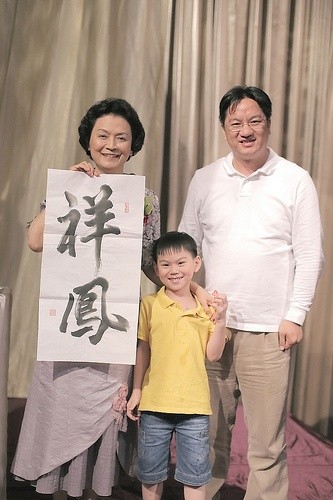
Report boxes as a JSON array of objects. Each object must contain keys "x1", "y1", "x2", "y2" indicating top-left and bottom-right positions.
[
  {"x1": 176, "y1": 85, "x2": 322, "y2": 500},
  {"x1": 6, "y1": 98, "x2": 160, "y2": 498},
  {"x1": 125, "y1": 231, "x2": 227, "y2": 500}
]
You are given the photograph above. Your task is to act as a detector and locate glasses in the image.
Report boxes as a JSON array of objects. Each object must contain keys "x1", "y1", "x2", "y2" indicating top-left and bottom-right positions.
[{"x1": 224, "y1": 116, "x2": 266, "y2": 132}]
